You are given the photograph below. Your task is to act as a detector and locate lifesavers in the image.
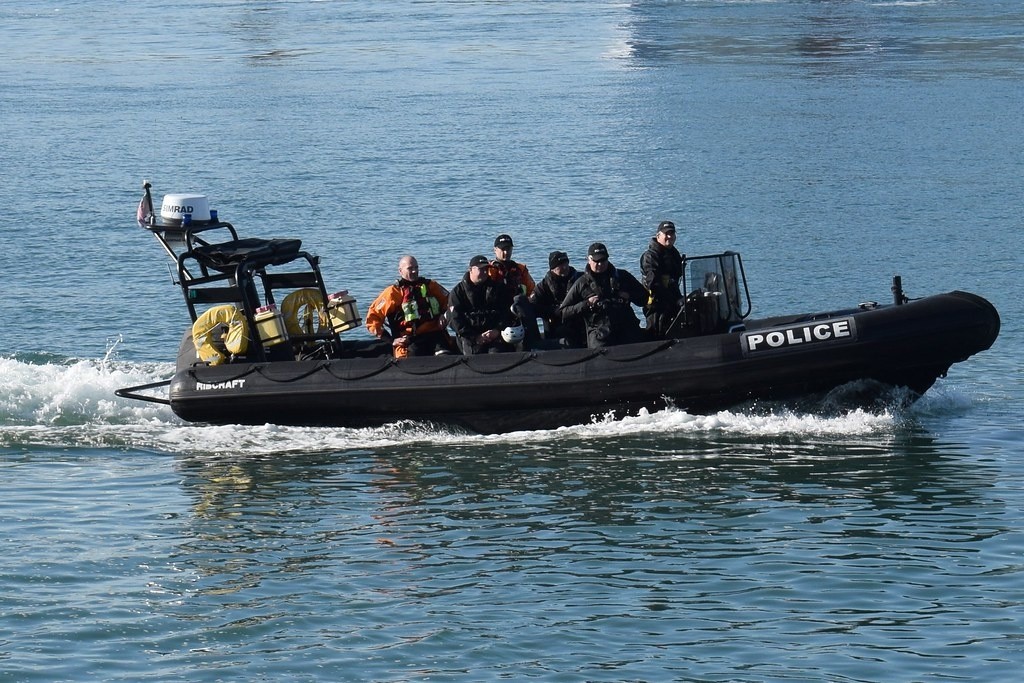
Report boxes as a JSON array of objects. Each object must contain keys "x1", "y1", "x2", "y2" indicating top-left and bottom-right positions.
[
  {"x1": 281, "y1": 289, "x2": 329, "y2": 336},
  {"x1": 192, "y1": 305, "x2": 248, "y2": 366},
  {"x1": 304, "y1": 303, "x2": 320, "y2": 347}
]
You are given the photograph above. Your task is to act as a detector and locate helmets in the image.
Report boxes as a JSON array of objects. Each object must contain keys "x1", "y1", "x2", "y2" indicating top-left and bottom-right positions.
[{"x1": 500, "y1": 319, "x2": 525, "y2": 343}]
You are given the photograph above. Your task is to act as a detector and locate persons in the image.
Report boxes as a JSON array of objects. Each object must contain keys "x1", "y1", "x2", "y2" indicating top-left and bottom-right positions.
[
  {"x1": 638, "y1": 221, "x2": 684, "y2": 329},
  {"x1": 446, "y1": 256, "x2": 512, "y2": 354},
  {"x1": 557, "y1": 242, "x2": 654, "y2": 350},
  {"x1": 486, "y1": 233, "x2": 546, "y2": 351},
  {"x1": 365, "y1": 255, "x2": 451, "y2": 358},
  {"x1": 523, "y1": 250, "x2": 588, "y2": 351}
]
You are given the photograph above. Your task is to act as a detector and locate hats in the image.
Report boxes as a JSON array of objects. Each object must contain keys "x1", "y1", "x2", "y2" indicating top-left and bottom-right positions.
[
  {"x1": 549, "y1": 251, "x2": 569, "y2": 270},
  {"x1": 588, "y1": 243, "x2": 609, "y2": 260},
  {"x1": 494, "y1": 235, "x2": 514, "y2": 248},
  {"x1": 470, "y1": 255, "x2": 494, "y2": 269},
  {"x1": 658, "y1": 221, "x2": 675, "y2": 233}
]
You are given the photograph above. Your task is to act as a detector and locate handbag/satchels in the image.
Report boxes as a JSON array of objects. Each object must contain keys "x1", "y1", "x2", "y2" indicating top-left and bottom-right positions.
[{"x1": 585, "y1": 301, "x2": 641, "y2": 348}]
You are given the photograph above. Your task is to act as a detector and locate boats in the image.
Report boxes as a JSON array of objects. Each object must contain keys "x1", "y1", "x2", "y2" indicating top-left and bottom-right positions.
[{"x1": 116, "y1": 181, "x2": 999, "y2": 428}]
